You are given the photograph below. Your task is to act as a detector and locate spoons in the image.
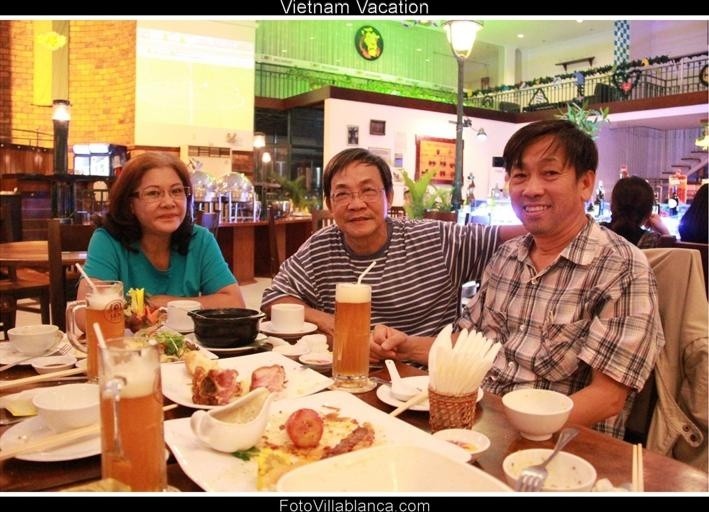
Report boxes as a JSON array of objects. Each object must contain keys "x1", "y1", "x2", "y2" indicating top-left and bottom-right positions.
[{"x1": 385, "y1": 358, "x2": 419, "y2": 399}]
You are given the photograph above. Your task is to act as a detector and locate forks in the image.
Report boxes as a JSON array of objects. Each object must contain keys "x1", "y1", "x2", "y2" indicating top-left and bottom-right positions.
[
  {"x1": 514, "y1": 427, "x2": 581, "y2": 492},
  {"x1": 0, "y1": 342, "x2": 87, "y2": 372}
]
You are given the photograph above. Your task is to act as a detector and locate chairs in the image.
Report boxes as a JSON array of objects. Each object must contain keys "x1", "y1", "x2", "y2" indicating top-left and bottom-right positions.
[
  {"x1": 660, "y1": 234, "x2": 708, "y2": 301},
  {"x1": 47, "y1": 215, "x2": 97, "y2": 333},
  {"x1": 0, "y1": 190, "x2": 49, "y2": 324},
  {"x1": 312, "y1": 207, "x2": 335, "y2": 233},
  {"x1": 388, "y1": 207, "x2": 405, "y2": 220}
]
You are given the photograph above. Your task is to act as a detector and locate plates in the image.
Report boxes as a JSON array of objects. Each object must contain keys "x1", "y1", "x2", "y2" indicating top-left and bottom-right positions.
[
  {"x1": 0, "y1": 341, "x2": 64, "y2": 364},
  {"x1": 377, "y1": 374, "x2": 484, "y2": 411},
  {"x1": 175, "y1": 332, "x2": 269, "y2": 350},
  {"x1": 160, "y1": 350, "x2": 337, "y2": 409},
  {"x1": 260, "y1": 321, "x2": 318, "y2": 334},
  {"x1": 163, "y1": 390, "x2": 471, "y2": 492},
  {"x1": 0, "y1": 402, "x2": 100, "y2": 461},
  {"x1": 3, "y1": 416, "x2": 100, "y2": 461},
  {"x1": 135, "y1": 326, "x2": 220, "y2": 358},
  {"x1": 276, "y1": 446, "x2": 515, "y2": 496},
  {"x1": 0, "y1": 327, "x2": 67, "y2": 365}
]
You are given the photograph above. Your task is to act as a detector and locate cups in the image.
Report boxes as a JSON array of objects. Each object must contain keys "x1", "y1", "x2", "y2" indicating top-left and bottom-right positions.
[
  {"x1": 66, "y1": 281, "x2": 126, "y2": 384},
  {"x1": 332, "y1": 283, "x2": 372, "y2": 390},
  {"x1": 167, "y1": 300, "x2": 201, "y2": 332},
  {"x1": 271, "y1": 303, "x2": 304, "y2": 334},
  {"x1": 99, "y1": 337, "x2": 165, "y2": 496}
]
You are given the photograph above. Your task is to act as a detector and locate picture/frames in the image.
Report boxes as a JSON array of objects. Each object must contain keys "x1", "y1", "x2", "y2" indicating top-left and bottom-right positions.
[
  {"x1": 370, "y1": 119, "x2": 386, "y2": 136},
  {"x1": 414, "y1": 135, "x2": 464, "y2": 185}
]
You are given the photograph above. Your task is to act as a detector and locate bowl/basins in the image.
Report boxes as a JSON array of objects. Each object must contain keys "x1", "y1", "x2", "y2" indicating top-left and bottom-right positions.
[
  {"x1": 187, "y1": 307, "x2": 266, "y2": 348},
  {"x1": 272, "y1": 340, "x2": 330, "y2": 356},
  {"x1": 33, "y1": 382, "x2": 107, "y2": 429},
  {"x1": 503, "y1": 448, "x2": 597, "y2": 491},
  {"x1": 433, "y1": 428, "x2": 491, "y2": 464},
  {"x1": 299, "y1": 350, "x2": 334, "y2": 367},
  {"x1": 502, "y1": 388, "x2": 574, "y2": 442},
  {"x1": 273, "y1": 344, "x2": 311, "y2": 356}
]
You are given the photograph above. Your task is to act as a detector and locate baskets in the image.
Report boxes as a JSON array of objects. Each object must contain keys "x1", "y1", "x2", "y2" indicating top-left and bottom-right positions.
[{"x1": 429, "y1": 391, "x2": 478, "y2": 433}]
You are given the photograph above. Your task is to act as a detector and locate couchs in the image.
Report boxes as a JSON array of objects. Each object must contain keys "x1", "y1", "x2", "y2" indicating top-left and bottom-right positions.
[{"x1": 523, "y1": 82, "x2": 618, "y2": 111}]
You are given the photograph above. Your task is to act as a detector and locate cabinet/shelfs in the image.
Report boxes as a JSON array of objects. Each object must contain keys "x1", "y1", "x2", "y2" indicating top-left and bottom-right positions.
[{"x1": 221, "y1": 220, "x2": 321, "y2": 285}]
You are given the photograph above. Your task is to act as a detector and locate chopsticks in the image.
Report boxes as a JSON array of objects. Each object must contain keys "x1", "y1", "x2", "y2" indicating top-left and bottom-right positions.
[
  {"x1": 1, "y1": 402, "x2": 180, "y2": 460},
  {"x1": 1, "y1": 365, "x2": 88, "y2": 392},
  {"x1": 388, "y1": 390, "x2": 429, "y2": 417},
  {"x1": 632, "y1": 443, "x2": 644, "y2": 494},
  {"x1": 390, "y1": 390, "x2": 444, "y2": 418}
]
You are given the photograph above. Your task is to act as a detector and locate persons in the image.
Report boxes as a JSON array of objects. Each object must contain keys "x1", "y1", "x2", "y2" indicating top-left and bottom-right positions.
[
  {"x1": 76, "y1": 151, "x2": 245, "y2": 334},
  {"x1": 372, "y1": 119, "x2": 664, "y2": 441},
  {"x1": 597, "y1": 176, "x2": 674, "y2": 253},
  {"x1": 265, "y1": 147, "x2": 527, "y2": 337}
]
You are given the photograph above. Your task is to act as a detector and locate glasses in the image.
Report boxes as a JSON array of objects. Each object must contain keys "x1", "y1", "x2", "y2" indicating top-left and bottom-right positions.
[
  {"x1": 129, "y1": 187, "x2": 193, "y2": 202},
  {"x1": 324, "y1": 185, "x2": 387, "y2": 202}
]
